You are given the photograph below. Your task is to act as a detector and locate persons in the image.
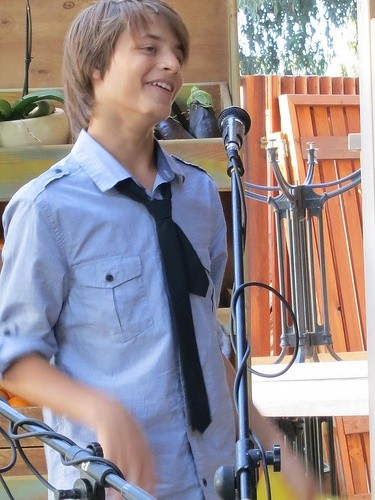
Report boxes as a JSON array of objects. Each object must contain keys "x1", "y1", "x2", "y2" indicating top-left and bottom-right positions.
[{"x1": 1, "y1": 0, "x2": 326, "y2": 500}]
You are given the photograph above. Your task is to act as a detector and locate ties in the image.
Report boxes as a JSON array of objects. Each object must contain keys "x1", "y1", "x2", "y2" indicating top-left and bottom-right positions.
[{"x1": 113, "y1": 177, "x2": 213, "y2": 436}]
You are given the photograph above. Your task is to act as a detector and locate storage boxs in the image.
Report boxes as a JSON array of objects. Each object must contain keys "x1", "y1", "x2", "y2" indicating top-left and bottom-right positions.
[
  {"x1": 143, "y1": 82, "x2": 239, "y2": 190},
  {"x1": 0, "y1": 86, "x2": 80, "y2": 203}
]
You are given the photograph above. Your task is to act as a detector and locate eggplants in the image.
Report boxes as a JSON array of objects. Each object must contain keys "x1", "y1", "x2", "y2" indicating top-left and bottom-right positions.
[{"x1": 155, "y1": 85, "x2": 220, "y2": 141}]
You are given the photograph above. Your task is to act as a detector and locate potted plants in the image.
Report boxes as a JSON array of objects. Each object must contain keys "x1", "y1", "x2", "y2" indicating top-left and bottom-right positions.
[{"x1": 0, "y1": 90, "x2": 69, "y2": 147}]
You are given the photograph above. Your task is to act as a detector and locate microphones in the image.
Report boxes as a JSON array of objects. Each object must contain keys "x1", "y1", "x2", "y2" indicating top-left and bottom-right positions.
[{"x1": 217, "y1": 106, "x2": 251, "y2": 160}]
